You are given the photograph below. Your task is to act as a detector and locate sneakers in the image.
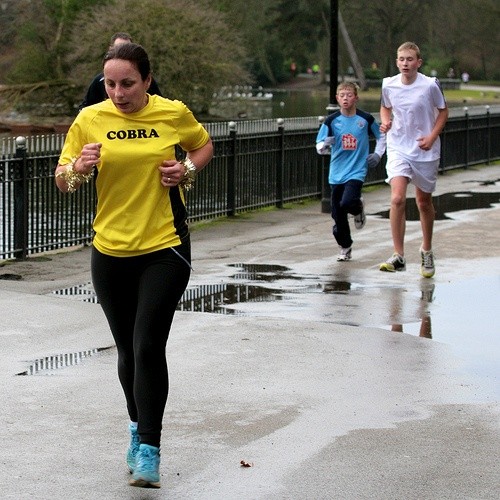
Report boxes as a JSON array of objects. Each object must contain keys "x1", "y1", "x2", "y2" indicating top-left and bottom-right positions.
[
  {"x1": 129, "y1": 443, "x2": 161, "y2": 487},
  {"x1": 125, "y1": 423, "x2": 141, "y2": 474},
  {"x1": 420, "y1": 279, "x2": 435, "y2": 303},
  {"x1": 354, "y1": 198, "x2": 366, "y2": 230},
  {"x1": 337, "y1": 246, "x2": 352, "y2": 261},
  {"x1": 379, "y1": 252, "x2": 406, "y2": 272},
  {"x1": 420, "y1": 246, "x2": 437, "y2": 278}
]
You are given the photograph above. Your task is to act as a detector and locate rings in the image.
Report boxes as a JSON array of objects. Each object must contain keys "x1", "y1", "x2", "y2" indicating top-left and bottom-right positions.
[
  {"x1": 167, "y1": 177, "x2": 171, "y2": 183},
  {"x1": 88, "y1": 155, "x2": 92, "y2": 160}
]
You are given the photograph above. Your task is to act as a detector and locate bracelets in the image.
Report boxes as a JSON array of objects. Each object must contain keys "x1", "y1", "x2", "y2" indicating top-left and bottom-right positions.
[
  {"x1": 179, "y1": 157, "x2": 196, "y2": 191},
  {"x1": 56, "y1": 157, "x2": 95, "y2": 194}
]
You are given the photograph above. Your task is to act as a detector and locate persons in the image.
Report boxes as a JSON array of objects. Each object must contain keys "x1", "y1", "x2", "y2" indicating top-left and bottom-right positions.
[
  {"x1": 379, "y1": 42, "x2": 448, "y2": 278},
  {"x1": 55, "y1": 42, "x2": 213, "y2": 489},
  {"x1": 316, "y1": 82, "x2": 386, "y2": 262},
  {"x1": 312, "y1": 61, "x2": 469, "y2": 85},
  {"x1": 77, "y1": 33, "x2": 164, "y2": 113}
]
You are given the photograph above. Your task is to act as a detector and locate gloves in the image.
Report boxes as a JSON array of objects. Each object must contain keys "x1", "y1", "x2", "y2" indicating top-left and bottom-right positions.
[
  {"x1": 323, "y1": 137, "x2": 334, "y2": 150},
  {"x1": 366, "y1": 153, "x2": 380, "y2": 168}
]
